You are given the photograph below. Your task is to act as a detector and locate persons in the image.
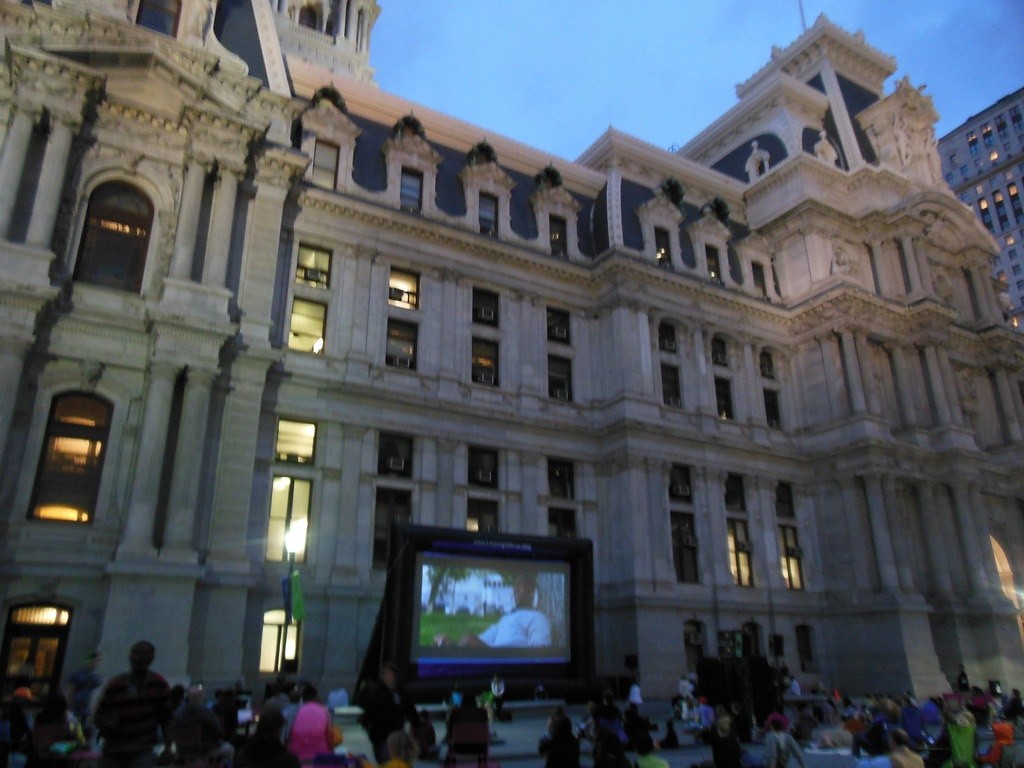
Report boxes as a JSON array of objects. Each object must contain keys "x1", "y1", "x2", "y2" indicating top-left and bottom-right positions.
[
  {"x1": 673, "y1": 663, "x2": 1024, "y2": 765},
  {"x1": 431, "y1": 566, "x2": 554, "y2": 654},
  {"x1": 4, "y1": 642, "x2": 666, "y2": 766},
  {"x1": 923, "y1": 127, "x2": 945, "y2": 186},
  {"x1": 893, "y1": 111, "x2": 913, "y2": 167}
]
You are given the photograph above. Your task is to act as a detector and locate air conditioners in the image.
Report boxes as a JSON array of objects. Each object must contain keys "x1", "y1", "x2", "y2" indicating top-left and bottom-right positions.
[
  {"x1": 304, "y1": 270, "x2": 321, "y2": 281},
  {"x1": 672, "y1": 485, "x2": 690, "y2": 498},
  {"x1": 391, "y1": 286, "x2": 404, "y2": 300},
  {"x1": 766, "y1": 367, "x2": 776, "y2": 379},
  {"x1": 475, "y1": 469, "x2": 496, "y2": 483},
  {"x1": 478, "y1": 374, "x2": 494, "y2": 385},
  {"x1": 739, "y1": 542, "x2": 751, "y2": 552},
  {"x1": 715, "y1": 353, "x2": 729, "y2": 367},
  {"x1": 664, "y1": 338, "x2": 680, "y2": 355},
  {"x1": 553, "y1": 482, "x2": 574, "y2": 497},
  {"x1": 552, "y1": 326, "x2": 568, "y2": 338},
  {"x1": 479, "y1": 309, "x2": 495, "y2": 321},
  {"x1": 789, "y1": 547, "x2": 805, "y2": 557},
  {"x1": 384, "y1": 457, "x2": 406, "y2": 471},
  {"x1": 554, "y1": 388, "x2": 568, "y2": 400},
  {"x1": 668, "y1": 398, "x2": 680, "y2": 408},
  {"x1": 771, "y1": 419, "x2": 780, "y2": 429},
  {"x1": 396, "y1": 356, "x2": 410, "y2": 367},
  {"x1": 683, "y1": 534, "x2": 698, "y2": 546}
]
